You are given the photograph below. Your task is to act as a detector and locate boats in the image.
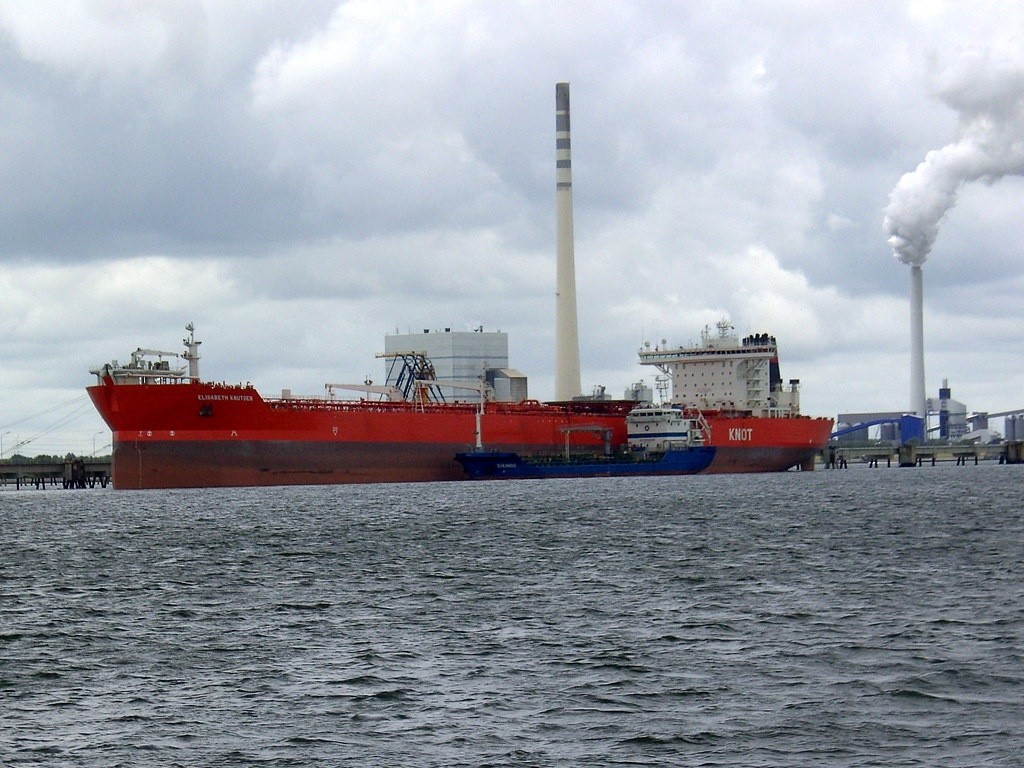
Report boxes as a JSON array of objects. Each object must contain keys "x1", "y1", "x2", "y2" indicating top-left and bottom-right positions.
[
  {"x1": 453, "y1": 370, "x2": 717, "y2": 479},
  {"x1": 86, "y1": 318, "x2": 834, "y2": 491}
]
